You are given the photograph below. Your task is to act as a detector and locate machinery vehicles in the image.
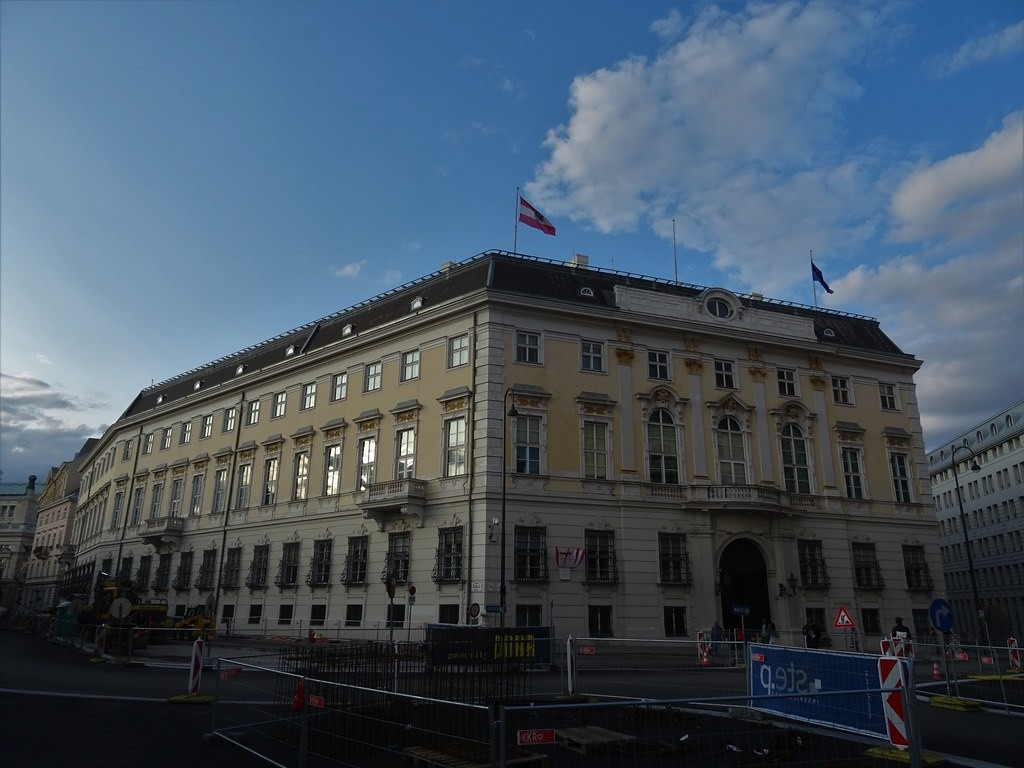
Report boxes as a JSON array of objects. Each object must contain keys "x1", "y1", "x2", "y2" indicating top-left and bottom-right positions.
[{"x1": 79, "y1": 573, "x2": 220, "y2": 646}]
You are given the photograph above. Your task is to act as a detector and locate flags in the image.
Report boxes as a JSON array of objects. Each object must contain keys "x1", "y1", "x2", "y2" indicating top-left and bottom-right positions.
[{"x1": 517, "y1": 196, "x2": 556, "y2": 234}]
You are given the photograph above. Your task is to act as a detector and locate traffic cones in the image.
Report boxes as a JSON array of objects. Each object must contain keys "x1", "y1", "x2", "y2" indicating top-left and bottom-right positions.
[
  {"x1": 703, "y1": 650, "x2": 710, "y2": 665},
  {"x1": 932, "y1": 660, "x2": 944, "y2": 680}
]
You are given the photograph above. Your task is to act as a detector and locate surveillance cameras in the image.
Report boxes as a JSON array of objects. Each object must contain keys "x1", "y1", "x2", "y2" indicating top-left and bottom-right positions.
[{"x1": 495, "y1": 520, "x2": 500, "y2": 525}]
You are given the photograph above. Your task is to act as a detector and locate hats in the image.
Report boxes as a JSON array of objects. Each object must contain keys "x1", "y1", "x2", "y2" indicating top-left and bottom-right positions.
[
  {"x1": 714, "y1": 618, "x2": 718, "y2": 621},
  {"x1": 895, "y1": 616, "x2": 903, "y2": 623}
]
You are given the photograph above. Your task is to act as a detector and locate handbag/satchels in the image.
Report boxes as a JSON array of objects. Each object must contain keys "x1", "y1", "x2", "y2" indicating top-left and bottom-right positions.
[{"x1": 707, "y1": 643, "x2": 712, "y2": 648}]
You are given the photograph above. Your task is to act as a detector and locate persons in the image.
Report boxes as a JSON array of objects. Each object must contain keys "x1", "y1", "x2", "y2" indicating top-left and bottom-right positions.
[
  {"x1": 892, "y1": 617, "x2": 911, "y2": 642},
  {"x1": 801, "y1": 617, "x2": 820, "y2": 648},
  {"x1": 761, "y1": 618, "x2": 768, "y2": 643},
  {"x1": 710, "y1": 621, "x2": 724, "y2": 658},
  {"x1": 767, "y1": 623, "x2": 780, "y2": 646}
]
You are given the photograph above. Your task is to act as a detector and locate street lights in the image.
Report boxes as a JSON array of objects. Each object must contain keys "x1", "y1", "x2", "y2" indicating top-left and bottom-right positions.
[
  {"x1": 951, "y1": 445, "x2": 987, "y2": 654},
  {"x1": 498, "y1": 385, "x2": 520, "y2": 634}
]
